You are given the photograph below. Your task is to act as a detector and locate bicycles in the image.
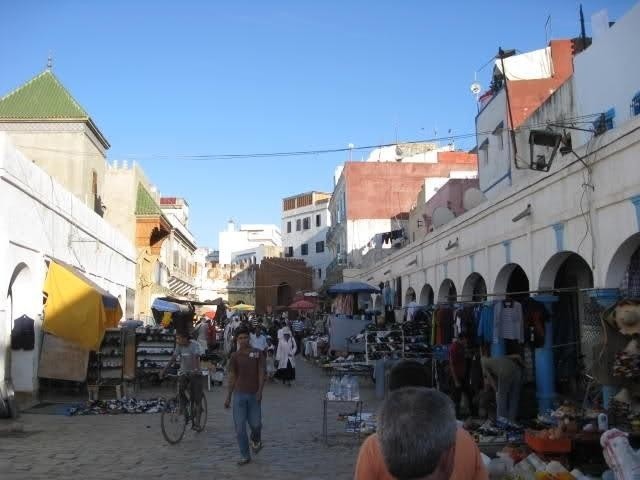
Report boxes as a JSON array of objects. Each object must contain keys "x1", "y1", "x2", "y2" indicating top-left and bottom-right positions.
[{"x1": 160, "y1": 370, "x2": 208, "y2": 445}]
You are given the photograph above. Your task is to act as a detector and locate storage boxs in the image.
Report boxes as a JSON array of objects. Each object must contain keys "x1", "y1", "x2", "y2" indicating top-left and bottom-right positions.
[{"x1": 524, "y1": 431, "x2": 572, "y2": 453}]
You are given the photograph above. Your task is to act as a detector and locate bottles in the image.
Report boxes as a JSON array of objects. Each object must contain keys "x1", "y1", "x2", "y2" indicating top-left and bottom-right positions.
[{"x1": 329, "y1": 374, "x2": 360, "y2": 400}]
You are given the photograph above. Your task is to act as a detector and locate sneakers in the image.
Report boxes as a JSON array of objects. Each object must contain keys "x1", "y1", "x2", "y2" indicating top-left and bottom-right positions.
[
  {"x1": 253, "y1": 442, "x2": 261, "y2": 451},
  {"x1": 238, "y1": 459, "x2": 248, "y2": 464}
]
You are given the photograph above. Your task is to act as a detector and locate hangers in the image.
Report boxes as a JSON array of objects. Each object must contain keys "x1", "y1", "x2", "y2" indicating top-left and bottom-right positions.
[{"x1": 433, "y1": 287, "x2": 575, "y2": 312}]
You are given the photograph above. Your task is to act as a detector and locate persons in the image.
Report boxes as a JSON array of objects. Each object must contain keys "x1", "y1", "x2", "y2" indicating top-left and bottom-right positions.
[
  {"x1": 206, "y1": 319, "x2": 216, "y2": 347},
  {"x1": 354, "y1": 358, "x2": 488, "y2": 480},
  {"x1": 190, "y1": 318, "x2": 208, "y2": 351},
  {"x1": 448, "y1": 331, "x2": 473, "y2": 408},
  {"x1": 223, "y1": 313, "x2": 312, "y2": 387},
  {"x1": 160, "y1": 330, "x2": 201, "y2": 432},
  {"x1": 481, "y1": 355, "x2": 526, "y2": 423},
  {"x1": 377, "y1": 386, "x2": 457, "y2": 480},
  {"x1": 224, "y1": 326, "x2": 265, "y2": 465}
]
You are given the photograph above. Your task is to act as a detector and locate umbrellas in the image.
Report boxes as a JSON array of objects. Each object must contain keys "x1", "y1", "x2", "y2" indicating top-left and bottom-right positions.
[
  {"x1": 232, "y1": 302, "x2": 255, "y2": 321},
  {"x1": 327, "y1": 282, "x2": 380, "y2": 296},
  {"x1": 288, "y1": 300, "x2": 316, "y2": 309}
]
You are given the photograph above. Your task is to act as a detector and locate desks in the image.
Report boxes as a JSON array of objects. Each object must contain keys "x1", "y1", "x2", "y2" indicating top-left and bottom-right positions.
[{"x1": 323, "y1": 398, "x2": 363, "y2": 446}]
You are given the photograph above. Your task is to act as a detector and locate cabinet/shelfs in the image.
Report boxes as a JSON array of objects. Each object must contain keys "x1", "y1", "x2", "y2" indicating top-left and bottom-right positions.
[
  {"x1": 97, "y1": 328, "x2": 178, "y2": 384},
  {"x1": 365, "y1": 328, "x2": 430, "y2": 371}
]
